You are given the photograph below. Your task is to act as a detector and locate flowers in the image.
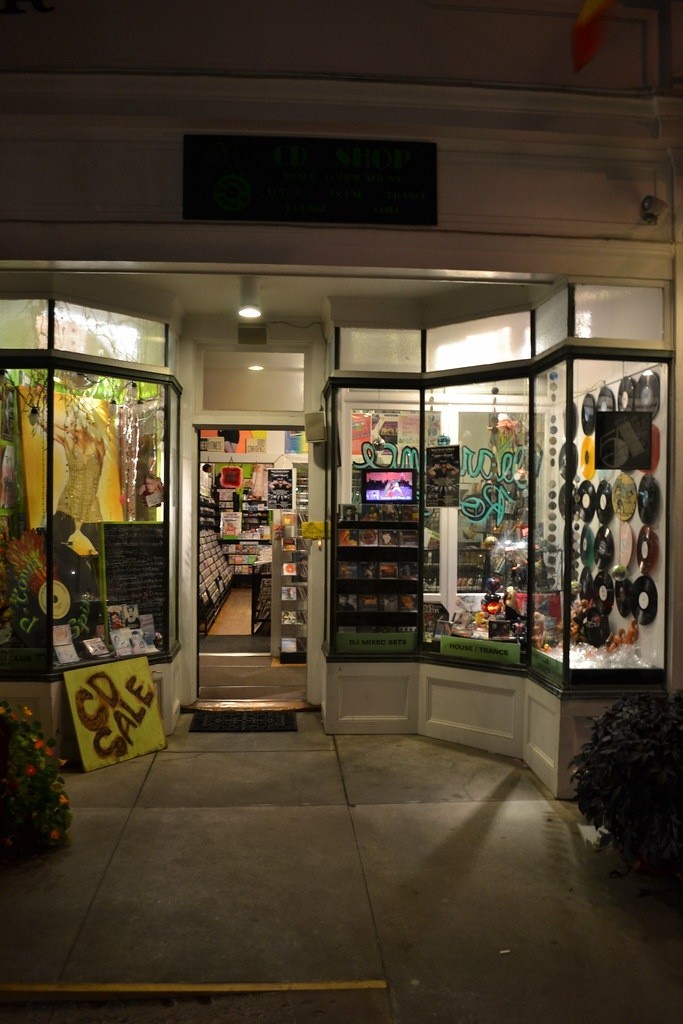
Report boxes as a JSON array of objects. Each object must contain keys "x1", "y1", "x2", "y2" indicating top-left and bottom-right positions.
[{"x1": 0, "y1": 699, "x2": 86, "y2": 850}]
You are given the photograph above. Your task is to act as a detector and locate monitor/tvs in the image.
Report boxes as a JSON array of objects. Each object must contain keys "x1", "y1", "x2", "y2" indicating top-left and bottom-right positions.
[{"x1": 361, "y1": 468, "x2": 418, "y2": 505}]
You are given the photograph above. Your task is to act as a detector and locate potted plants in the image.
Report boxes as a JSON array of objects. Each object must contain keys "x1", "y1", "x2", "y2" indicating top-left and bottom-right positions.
[{"x1": 568, "y1": 690, "x2": 683, "y2": 877}]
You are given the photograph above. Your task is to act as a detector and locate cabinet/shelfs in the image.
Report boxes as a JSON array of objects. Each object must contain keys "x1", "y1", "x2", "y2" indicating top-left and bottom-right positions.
[
  {"x1": 334, "y1": 516, "x2": 421, "y2": 629},
  {"x1": 278, "y1": 534, "x2": 307, "y2": 665}
]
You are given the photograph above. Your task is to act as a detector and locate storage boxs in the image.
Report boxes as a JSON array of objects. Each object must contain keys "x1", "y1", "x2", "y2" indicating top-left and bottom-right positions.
[{"x1": 199, "y1": 436, "x2": 268, "y2": 454}]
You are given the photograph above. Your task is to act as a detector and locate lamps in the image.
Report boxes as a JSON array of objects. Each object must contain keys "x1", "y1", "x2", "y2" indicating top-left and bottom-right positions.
[
  {"x1": 0, "y1": 368, "x2": 165, "y2": 426},
  {"x1": 304, "y1": 413, "x2": 329, "y2": 443},
  {"x1": 239, "y1": 276, "x2": 262, "y2": 318}
]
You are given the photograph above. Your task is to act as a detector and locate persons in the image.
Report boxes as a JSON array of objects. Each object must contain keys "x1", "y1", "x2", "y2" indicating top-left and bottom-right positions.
[
  {"x1": 34, "y1": 413, "x2": 106, "y2": 522},
  {"x1": 112, "y1": 612, "x2": 124, "y2": 630},
  {"x1": 498, "y1": 587, "x2": 638, "y2": 652},
  {"x1": 139, "y1": 476, "x2": 162, "y2": 505},
  {"x1": 386, "y1": 481, "x2": 404, "y2": 497},
  {"x1": 269, "y1": 479, "x2": 291, "y2": 488},
  {"x1": 125, "y1": 606, "x2": 141, "y2": 630}
]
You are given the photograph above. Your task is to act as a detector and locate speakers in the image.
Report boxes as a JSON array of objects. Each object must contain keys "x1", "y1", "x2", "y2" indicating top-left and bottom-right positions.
[
  {"x1": 238, "y1": 324, "x2": 268, "y2": 345},
  {"x1": 305, "y1": 411, "x2": 327, "y2": 442}
]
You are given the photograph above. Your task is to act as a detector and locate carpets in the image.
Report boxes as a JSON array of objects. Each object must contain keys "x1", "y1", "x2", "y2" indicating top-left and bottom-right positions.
[{"x1": 189, "y1": 710, "x2": 298, "y2": 733}]
